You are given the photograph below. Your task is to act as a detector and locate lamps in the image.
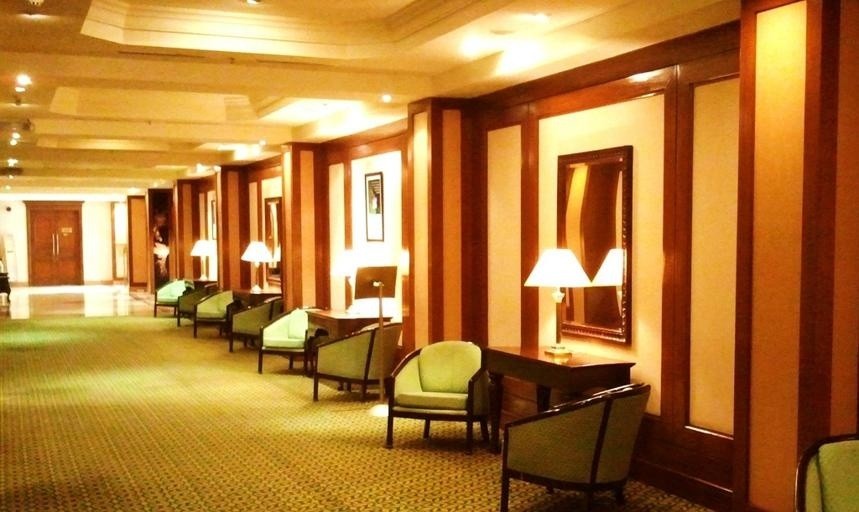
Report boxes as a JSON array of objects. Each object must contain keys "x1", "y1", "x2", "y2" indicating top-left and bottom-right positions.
[
  {"x1": 524, "y1": 250, "x2": 595, "y2": 360},
  {"x1": 191, "y1": 239, "x2": 215, "y2": 280},
  {"x1": 242, "y1": 240, "x2": 274, "y2": 293},
  {"x1": 593, "y1": 249, "x2": 625, "y2": 318}
]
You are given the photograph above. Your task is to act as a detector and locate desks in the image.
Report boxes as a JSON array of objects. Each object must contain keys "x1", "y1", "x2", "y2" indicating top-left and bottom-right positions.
[
  {"x1": 231, "y1": 289, "x2": 281, "y2": 302},
  {"x1": 486, "y1": 346, "x2": 636, "y2": 454},
  {"x1": 306, "y1": 309, "x2": 393, "y2": 388},
  {"x1": 179, "y1": 278, "x2": 210, "y2": 289}
]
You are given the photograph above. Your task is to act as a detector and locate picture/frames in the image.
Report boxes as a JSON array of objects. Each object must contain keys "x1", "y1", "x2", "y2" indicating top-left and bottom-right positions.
[{"x1": 364, "y1": 171, "x2": 384, "y2": 242}]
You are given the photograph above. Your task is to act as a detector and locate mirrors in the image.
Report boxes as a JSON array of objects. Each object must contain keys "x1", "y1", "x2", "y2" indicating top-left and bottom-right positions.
[{"x1": 556, "y1": 146, "x2": 632, "y2": 347}]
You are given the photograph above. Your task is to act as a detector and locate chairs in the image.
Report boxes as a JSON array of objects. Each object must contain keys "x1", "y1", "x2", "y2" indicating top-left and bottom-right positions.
[
  {"x1": 794, "y1": 435, "x2": 859, "y2": 512},
  {"x1": 258, "y1": 306, "x2": 327, "y2": 375},
  {"x1": 194, "y1": 290, "x2": 244, "y2": 336},
  {"x1": 499, "y1": 383, "x2": 651, "y2": 512},
  {"x1": 176, "y1": 284, "x2": 222, "y2": 328},
  {"x1": 385, "y1": 341, "x2": 489, "y2": 453},
  {"x1": 227, "y1": 293, "x2": 284, "y2": 352},
  {"x1": 154, "y1": 279, "x2": 182, "y2": 316}
]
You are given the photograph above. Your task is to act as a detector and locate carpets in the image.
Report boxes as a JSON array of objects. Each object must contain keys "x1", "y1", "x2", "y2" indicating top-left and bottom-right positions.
[{"x1": 0, "y1": 319, "x2": 734, "y2": 512}]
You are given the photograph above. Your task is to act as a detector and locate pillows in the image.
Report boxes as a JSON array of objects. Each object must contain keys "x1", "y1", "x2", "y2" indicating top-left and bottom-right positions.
[
  {"x1": 292, "y1": 308, "x2": 312, "y2": 338},
  {"x1": 417, "y1": 343, "x2": 481, "y2": 392},
  {"x1": 816, "y1": 442, "x2": 856, "y2": 510}
]
